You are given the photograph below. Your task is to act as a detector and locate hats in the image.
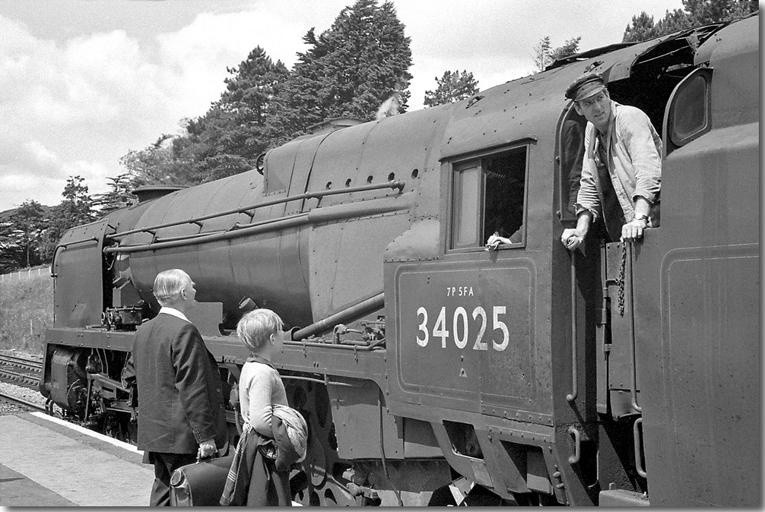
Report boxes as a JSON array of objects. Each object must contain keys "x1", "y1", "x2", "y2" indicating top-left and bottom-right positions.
[{"x1": 564, "y1": 72, "x2": 605, "y2": 102}]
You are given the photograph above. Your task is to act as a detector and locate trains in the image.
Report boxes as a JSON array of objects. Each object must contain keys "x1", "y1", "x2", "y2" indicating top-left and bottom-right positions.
[{"x1": 40, "y1": 10, "x2": 765, "y2": 507}]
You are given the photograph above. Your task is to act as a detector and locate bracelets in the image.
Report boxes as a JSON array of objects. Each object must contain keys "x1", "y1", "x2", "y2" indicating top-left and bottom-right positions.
[{"x1": 632, "y1": 211, "x2": 649, "y2": 221}]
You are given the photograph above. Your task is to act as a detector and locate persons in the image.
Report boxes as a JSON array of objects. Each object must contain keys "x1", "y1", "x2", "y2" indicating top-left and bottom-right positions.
[
  {"x1": 554, "y1": 74, "x2": 663, "y2": 243},
  {"x1": 237, "y1": 308, "x2": 290, "y2": 505},
  {"x1": 484, "y1": 120, "x2": 584, "y2": 253},
  {"x1": 122, "y1": 268, "x2": 227, "y2": 508}
]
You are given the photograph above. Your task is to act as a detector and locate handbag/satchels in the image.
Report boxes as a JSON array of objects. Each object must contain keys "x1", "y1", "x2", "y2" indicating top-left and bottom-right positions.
[{"x1": 169, "y1": 447, "x2": 237, "y2": 507}]
